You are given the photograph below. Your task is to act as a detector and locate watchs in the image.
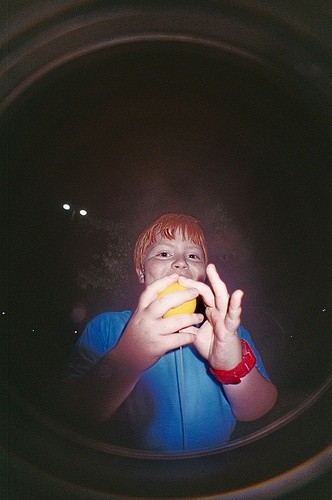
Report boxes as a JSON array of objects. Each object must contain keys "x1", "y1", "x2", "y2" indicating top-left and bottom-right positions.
[{"x1": 209, "y1": 339, "x2": 257, "y2": 384}]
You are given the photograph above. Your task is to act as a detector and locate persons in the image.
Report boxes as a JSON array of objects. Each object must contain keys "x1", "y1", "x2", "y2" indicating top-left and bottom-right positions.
[{"x1": 61, "y1": 212, "x2": 279, "y2": 498}]
